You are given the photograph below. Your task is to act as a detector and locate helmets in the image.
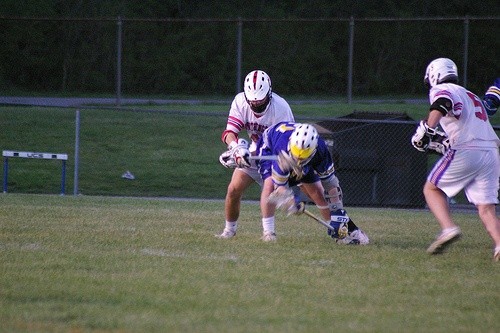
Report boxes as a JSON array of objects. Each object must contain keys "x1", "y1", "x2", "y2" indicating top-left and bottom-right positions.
[
  {"x1": 288, "y1": 124, "x2": 318, "y2": 166},
  {"x1": 244, "y1": 70, "x2": 272, "y2": 116},
  {"x1": 424, "y1": 58, "x2": 458, "y2": 86}
]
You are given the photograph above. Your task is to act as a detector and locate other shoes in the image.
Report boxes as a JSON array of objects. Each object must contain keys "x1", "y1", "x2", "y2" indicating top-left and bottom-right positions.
[
  {"x1": 427, "y1": 227, "x2": 460, "y2": 254},
  {"x1": 494, "y1": 248, "x2": 500, "y2": 261},
  {"x1": 262, "y1": 231, "x2": 276, "y2": 241},
  {"x1": 350, "y1": 229, "x2": 369, "y2": 245},
  {"x1": 337, "y1": 237, "x2": 360, "y2": 245},
  {"x1": 220, "y1": 229, "x2": 236, "y2": 238}
]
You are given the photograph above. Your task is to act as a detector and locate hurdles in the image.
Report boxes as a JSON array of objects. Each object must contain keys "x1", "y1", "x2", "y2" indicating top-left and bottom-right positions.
[{"x1": 2, "y1": 150, "x2": 68, "y2": 196}]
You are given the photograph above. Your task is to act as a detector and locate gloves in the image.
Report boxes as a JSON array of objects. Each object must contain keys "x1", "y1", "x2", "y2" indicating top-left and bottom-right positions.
[
  {"x1": 326, "y1": 209, "x2": 349, "y2": 239},
  {"x1": 229, "y1": 145, "x2": 252, "y2": 168},
  {"x1": 437, "y1": 138, "x2": 450, "y2": 154},
  {"x1": 411, "y1": 120, "x2": 438, "y2": 153}
]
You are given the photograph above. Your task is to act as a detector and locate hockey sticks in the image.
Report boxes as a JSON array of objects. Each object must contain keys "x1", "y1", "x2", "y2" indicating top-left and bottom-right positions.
[
  {"x1": 246, "y1": 150, "x2": 302, "y2": 178},
  {"x1": 269, "y1": 187, "x2": 335, "y2": 231}
]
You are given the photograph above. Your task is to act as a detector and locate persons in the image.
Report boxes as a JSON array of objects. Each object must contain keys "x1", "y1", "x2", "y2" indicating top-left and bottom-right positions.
[
  {"x1": 214, "y1": 70, "x2": 369, "y2": 246},
  {"x1": 255, "y1": 122, "x2": 360, "y2": 246},
  {"x1": 411, "y1": 58, "x2": 500, "y2": 261}
]
